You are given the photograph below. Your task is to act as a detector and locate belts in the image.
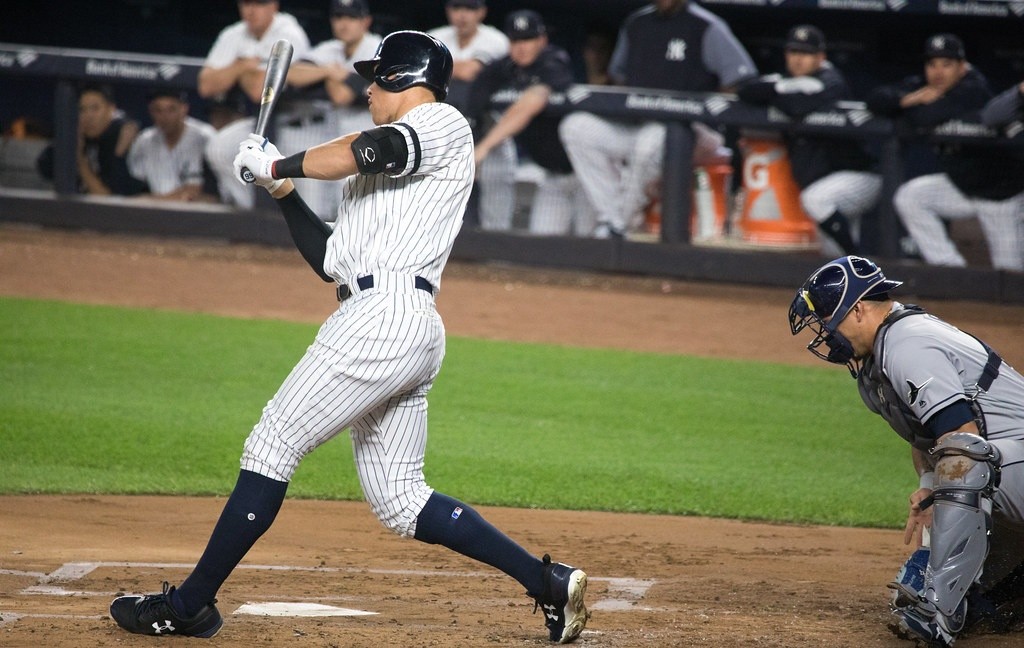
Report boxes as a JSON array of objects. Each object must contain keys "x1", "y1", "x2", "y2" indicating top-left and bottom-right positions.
[{"x1": 335, "y1": 276, "x2": 433, "y2": 300}]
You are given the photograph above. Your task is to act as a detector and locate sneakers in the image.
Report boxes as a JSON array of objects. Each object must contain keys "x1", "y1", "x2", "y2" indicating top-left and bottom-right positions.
[
  {"x1": 111, "y1": 581, "x2": 223, "y2": 639},
  {"x1": 892, "y1": 608, "x2": 951, "y2": 648},
  {"x1": 524, "y1": 554, "x2": 588, "y2": 644}
]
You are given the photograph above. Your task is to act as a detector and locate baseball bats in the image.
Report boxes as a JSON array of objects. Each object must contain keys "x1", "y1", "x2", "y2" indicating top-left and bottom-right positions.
[{"x1": 239, "y1": 38, "x2": 295, "y2": 184}]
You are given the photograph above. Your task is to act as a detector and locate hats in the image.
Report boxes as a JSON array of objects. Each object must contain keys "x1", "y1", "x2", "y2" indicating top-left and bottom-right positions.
[
  {"x1": 507, "y1": 9, "x2": 548, "y2": 38},
  {"x1": 921, "y1": 35, "x2": 968, "y2": 62},
  {"x1": 328, "y1": 0, "x2": 370, "y2": 18},
  {"x1": 784, "y1": 26, "x2": 827, "y2": 52}
]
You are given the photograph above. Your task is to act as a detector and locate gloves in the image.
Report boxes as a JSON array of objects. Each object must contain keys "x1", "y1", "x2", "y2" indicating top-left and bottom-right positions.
[
  {"x1": 233, "y1": 147, "x2": 274, "y2": 186},
  {"x1": 239, "y1": 135, "x2": 287, "y2": 194}
]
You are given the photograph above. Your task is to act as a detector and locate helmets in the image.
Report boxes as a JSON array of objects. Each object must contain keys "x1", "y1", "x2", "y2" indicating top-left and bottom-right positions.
[
  {"x1": 788, "y1": 255, "x2": 905, "y2": 378},
  {"x1": 353, "y1": 29, "x2": 453, "y2": 102}
]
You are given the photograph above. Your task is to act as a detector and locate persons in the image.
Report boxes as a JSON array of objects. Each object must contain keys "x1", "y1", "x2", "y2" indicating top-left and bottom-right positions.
[
  {"x1": 198, "y1": 0, "x2": 594, "y2": 237},
  {"x1": 123, "y1": 89, "x2": 215, "y2": 204},
  {"x1": 978, "y1": 81, "x2": 1024, "y2": 128},
  {"x1": 77, "y1": 89, "x2": 149, "y2": 198},
  {"x1": 558, "y1": 0, "x2": 756, "y2": 239},
  {"x1": 788, "y1": 256, "x2": 1024, "y2": 648},
  {"x1": 108, "y1": 31, "x2": 589, "y2": 645},
  {"x1": 868, "y1": 31, "x2": 1024, "y2": 270},
  {"x1": 0, "y1": 75, "x2": 26, "y2": 138},
  {"x1": 747, "y1": 24, "x2": 887, "y2": 256}
]
